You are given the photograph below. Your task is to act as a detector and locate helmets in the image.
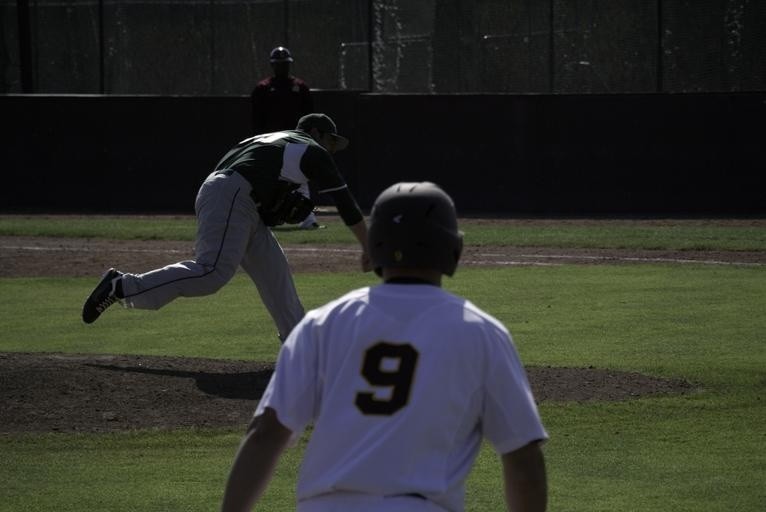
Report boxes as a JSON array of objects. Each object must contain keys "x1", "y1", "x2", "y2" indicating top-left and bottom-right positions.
[{"x1": 360, "y1": 180, "x2": 465, "y2": 277}]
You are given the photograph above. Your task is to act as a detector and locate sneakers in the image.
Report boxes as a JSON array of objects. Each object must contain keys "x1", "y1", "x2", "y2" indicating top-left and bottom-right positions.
[{"x1": 82, "y1": 268, "x2": 124, "y2": 324}]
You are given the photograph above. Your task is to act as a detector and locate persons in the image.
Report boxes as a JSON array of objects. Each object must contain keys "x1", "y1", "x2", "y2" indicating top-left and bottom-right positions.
[
  {"x1": 82, "y1": 113, "x2": 374, "y2": 345},
  {"x1": 251, "y1": 46, "x2": 327, "y2": 230},
  {"x1": 222, "y1": 181, "x2": 549, "y2": 512}
]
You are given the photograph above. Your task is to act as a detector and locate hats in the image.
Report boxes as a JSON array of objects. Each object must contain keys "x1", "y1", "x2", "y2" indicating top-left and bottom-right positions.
[
  {"x1": 269, "y1": 46, "x2": 294, "y2": 64},
  {"x1": 296, "y1": 113, "x2": 350, "y2": 151}
]
